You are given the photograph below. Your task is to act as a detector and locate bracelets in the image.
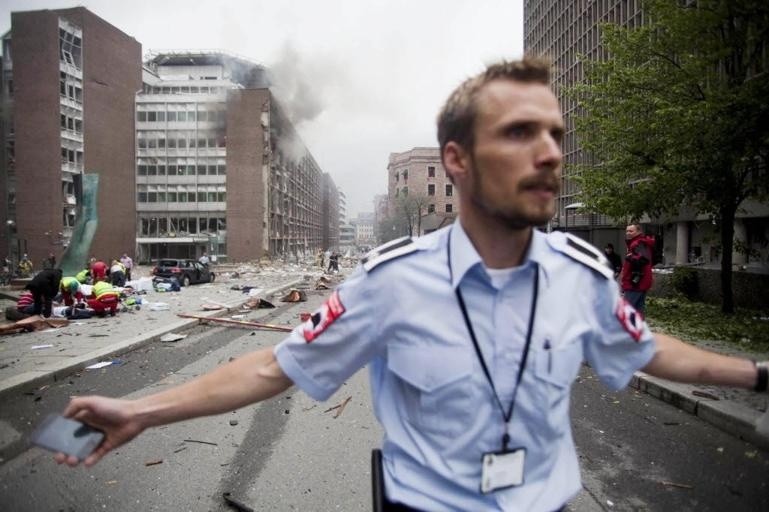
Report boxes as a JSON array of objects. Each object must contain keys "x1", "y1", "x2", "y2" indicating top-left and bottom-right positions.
[{"x1": 749, "y1": 357, "x2": 768, "y2": 395}]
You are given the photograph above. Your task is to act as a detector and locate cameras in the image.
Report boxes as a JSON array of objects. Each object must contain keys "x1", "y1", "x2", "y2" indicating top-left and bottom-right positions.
[{"x1": 631, "y1": 272, "x2": 642, "y2": 286}]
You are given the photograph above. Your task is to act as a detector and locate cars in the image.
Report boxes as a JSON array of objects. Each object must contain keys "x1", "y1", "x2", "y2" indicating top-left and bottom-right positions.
[
  {"x1": 357, "y1": 245, "x2": 371, "y2": 253},
  {"x1": 153, "y1": 259, "x2": 215, "y2": 287}
]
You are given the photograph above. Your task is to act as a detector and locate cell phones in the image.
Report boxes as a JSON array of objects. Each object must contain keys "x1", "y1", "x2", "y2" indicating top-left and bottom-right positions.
[{"x1": 30, "y1": 413, "x2": 106, "y2": 462}]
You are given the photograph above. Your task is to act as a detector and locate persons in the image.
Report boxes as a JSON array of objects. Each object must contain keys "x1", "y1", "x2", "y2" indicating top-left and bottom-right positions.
[
  {"x1": 2, "y1": 252, "x2": 135, "y2": 319},
  {"x1": 318, "y1": 246, "x2": 376, "y2": 273},
  {"x1": 604, "y1": 242, "x2": 623, "y2": 280},
  {"x1": 198, "y1": 252, "x2": 209, "y2": 265},
  {"x1": 616, "y1": 221, "x2": 657, "y2": 319},
  {"x1": 165, "y1": 275, "x2": 180, "y2": 292},
  {"x1": 52, "y1": 55, "x2": 768, "y2": 510}
]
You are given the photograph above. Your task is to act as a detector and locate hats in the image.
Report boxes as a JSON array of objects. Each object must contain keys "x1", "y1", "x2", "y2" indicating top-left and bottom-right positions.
[{"x1": 604, "y1": 242, "x2": 615, "y2": 249}]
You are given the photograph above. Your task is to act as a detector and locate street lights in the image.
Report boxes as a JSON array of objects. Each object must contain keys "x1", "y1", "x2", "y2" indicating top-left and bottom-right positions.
[
  {"x1": 6, "y1": 220, "x2": 15, "y2": 273},
  {"x1": 564, "y1": 202, "x2": 586, "y2": 232}
]
